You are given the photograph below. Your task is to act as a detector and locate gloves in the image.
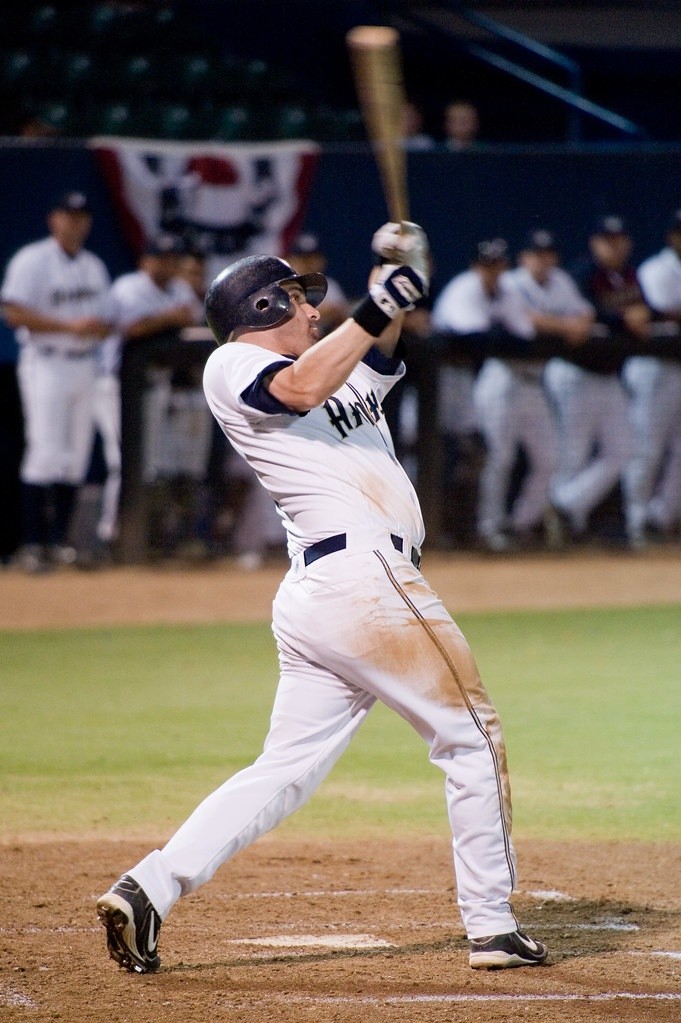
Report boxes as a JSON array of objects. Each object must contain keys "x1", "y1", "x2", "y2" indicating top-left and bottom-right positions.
[
  {"x1": 371, "y1": 220, "x2": 431, "y2": 296},
  {"x1": 368, "y1": 263, "x2": 429, "y2": 319}
]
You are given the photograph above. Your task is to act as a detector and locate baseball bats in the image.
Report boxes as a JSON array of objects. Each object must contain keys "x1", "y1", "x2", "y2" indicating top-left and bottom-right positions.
[{"x1": 350, "y1": 25, "x2": 409, "y2": 232}]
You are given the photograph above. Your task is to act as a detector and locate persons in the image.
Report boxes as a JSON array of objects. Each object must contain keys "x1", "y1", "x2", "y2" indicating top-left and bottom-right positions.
[
  {"x1": 365, "y1": 102, "x2": 482, "y2": 151},
  {"x1": 0, "y1": 191, "x2": 428, "y2": 574},
  {"x1": 96, "y1": 221, "x2": 548, "y2": 974},
  {"x1": 430, "y1": 213, "x2": 681, "y2": 552}
]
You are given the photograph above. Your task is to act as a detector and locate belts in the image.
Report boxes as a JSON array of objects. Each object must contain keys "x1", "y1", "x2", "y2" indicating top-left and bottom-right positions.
[
  {"x1": 42, "y1": 346, "x2": 95, "y2": 359},
  {"x1": 305, "y1": 534, "x2": 422, "y2": 571}
]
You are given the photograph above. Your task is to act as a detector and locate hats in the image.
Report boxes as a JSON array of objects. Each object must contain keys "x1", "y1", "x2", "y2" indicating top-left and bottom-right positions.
[
  {"x1": 588, "y1": 216, "x2": 631, "y2": 235},
  {"x1": 294, "y1": 231, "x2": 326, "y2": 255},
  {"x1": 52, "y1": 190, "x2": 91, "y2": 214},
  {"x1": 477, "y1": 232, "x2": 511, "y2": 264},
  {"x1": 141, "y1": 233, "x2": 184, "y2": 253},
  {"x1": 523, "y1": 224, "x2": 563, "y2": 252},
  {"x1": 189, "y1": 231, "x2": 222, "y2": 260},
  {"x1": 670, "y1": 209, "x2": 681, "y2": 228}
]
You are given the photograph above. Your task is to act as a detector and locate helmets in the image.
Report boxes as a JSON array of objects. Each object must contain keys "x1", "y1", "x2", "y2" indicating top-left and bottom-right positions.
[{"x1": 203, "y1": 255, "x2": 328, "y2": 346}]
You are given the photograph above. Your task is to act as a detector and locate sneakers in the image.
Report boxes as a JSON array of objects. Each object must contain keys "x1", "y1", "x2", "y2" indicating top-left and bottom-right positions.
[
  {"x1": 470, "y1": 930, "x2": 550, "y2": 969},
  {"x1": 98, "y1": 876, "x2": 162, "y2": 973}
]
